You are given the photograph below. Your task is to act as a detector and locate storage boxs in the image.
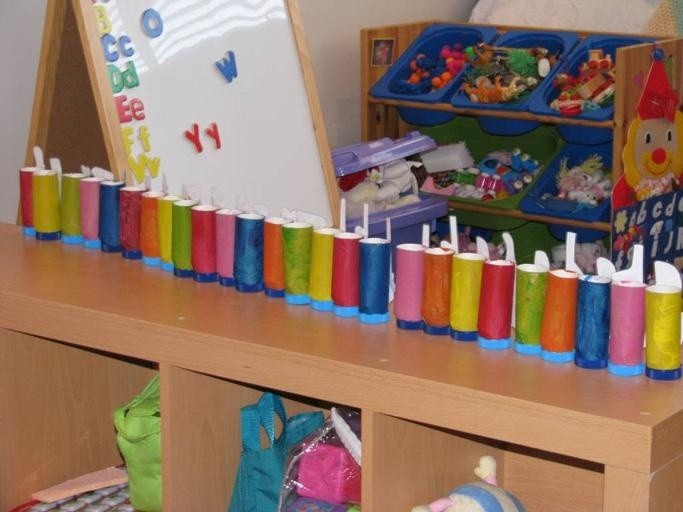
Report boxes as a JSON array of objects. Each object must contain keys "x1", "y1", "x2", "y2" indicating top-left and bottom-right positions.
[
  {"x1": 452, "y1": 29, "x2": 578, "y2": 137},
  {"x1": 369, "y1": 23, "x2": 498, "y2": 127},
  {"x1": 405, "y1": 117, "x2": 566, "y2": 232},
  {"x1": 520, "y1": 143, "x2": 614, "y2": 243},
  {"x1": 528, "y1": 32, "x2": 659, "y2": 145},
  {"x1": 330, "y1": 131, "x2": 448, "y2": 271}
]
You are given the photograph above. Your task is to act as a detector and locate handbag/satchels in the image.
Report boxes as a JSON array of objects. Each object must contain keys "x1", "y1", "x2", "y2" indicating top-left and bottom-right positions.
[
  {"x1": 229, "y1": 393, "x2": 323, "y2": 511},
  {"x1": 114, "y1": 374, "x2": 162, "y2": 512}
]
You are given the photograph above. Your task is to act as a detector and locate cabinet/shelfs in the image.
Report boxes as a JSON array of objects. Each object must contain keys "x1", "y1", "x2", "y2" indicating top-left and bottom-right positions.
[
  {"x1": 0, "y1": 222, "x2": 683, "y2": 511},
  {"x1": 360, "y1": 19, "x2": 683, "y2": 275}
]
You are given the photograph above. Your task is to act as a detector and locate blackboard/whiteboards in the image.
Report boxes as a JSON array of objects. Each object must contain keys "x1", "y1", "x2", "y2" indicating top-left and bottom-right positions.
[{"x1": 15, "y1": 0, "x2": 341, "y2": 227}]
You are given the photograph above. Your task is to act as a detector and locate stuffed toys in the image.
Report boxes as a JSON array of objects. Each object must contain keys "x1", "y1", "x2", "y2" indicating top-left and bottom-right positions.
[
  {"x1": 410, "y1": 455, "x2": 524, "y2": 512},
  {"x1": 557, "y1": 154, "x2": 615, "y2": 207},
  {"x1": 444, "y1": 234, "x2": 610, "y2": 272},
  {"x1": 390, "y1": 41, "x2": 472, "y2": 96},
  {"x1": 337, "y1": 158, "x2": 423, "y2": 218}
]
